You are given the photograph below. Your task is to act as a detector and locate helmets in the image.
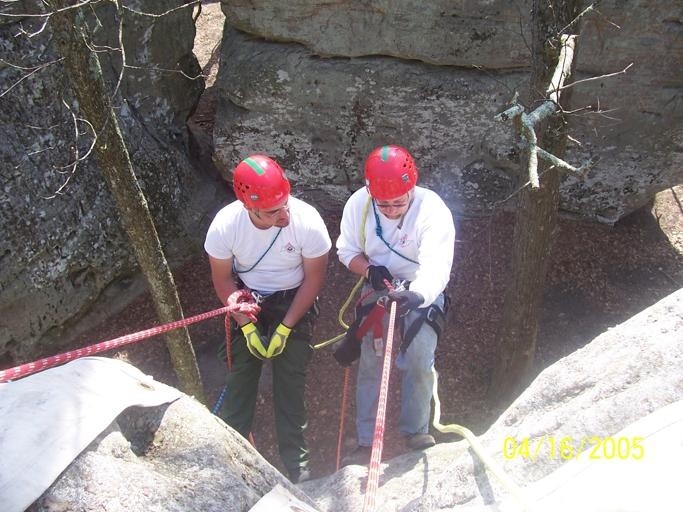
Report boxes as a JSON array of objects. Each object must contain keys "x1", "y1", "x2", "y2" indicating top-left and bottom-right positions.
[
  {"x1": 364, "y1": 144, "x2": 418, "y2": 200},
  {"x1": 233, "y1": 155, "x2": 290, "y2": 208}
]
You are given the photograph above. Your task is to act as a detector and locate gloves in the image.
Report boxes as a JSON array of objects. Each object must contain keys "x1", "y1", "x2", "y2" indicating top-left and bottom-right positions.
[
  {"x1": 385, "y1": 291, "x2": 425, "y2": 318},
  {"x1": 365, "y1": 264, "x2": 393, "y2": 291},
  {"x1": 240, "y1": 321, "x2": 292, "y2": 360}
]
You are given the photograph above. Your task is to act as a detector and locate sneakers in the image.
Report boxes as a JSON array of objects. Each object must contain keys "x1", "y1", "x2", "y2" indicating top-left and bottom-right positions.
[
  {"x1": 343, "y1": 446, "x2": 372, "y2": 466},
  {"x1": 406, "y1": 433, "x2": 435, "y2": 449},
  {"x1": 292, "y1": 467, "x2": 313, "y2": 482}
]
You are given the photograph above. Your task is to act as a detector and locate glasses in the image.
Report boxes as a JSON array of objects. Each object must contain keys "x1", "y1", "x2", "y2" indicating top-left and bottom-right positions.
[
  {"x1": 264, "y1": 204, "x2": 290, "y2": 218},
  {"x1": 373, "y1": 204, "x2": 409, "y2": 208}
]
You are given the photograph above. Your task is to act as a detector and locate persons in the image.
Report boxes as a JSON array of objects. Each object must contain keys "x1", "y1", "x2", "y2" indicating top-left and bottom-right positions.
[
  {"x1": 333, "y1": 145, "x2": 458, "y2": 467},
  {"x1": 203, "y1": 152, "x2": 333, "y2": 482}
]
